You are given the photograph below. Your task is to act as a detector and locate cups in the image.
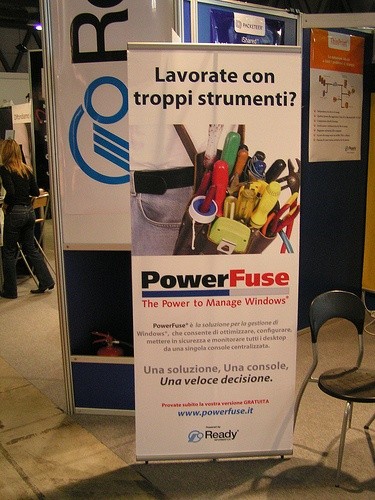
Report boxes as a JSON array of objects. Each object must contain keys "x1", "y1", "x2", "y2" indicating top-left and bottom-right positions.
[{"x1": 249, "y1": 230, "x2": 276, "y2": 254}]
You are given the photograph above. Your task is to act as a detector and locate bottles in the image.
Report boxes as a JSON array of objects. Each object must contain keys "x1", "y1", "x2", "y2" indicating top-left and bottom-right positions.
[{"x1": 173, "y1": 195, "x2": 218, "y2": 254}]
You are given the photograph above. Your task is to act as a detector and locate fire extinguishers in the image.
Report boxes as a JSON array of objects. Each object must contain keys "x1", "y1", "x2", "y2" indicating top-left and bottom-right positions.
[{"x1": 92, "y1": 331, "x2": 134, "y2": 357}]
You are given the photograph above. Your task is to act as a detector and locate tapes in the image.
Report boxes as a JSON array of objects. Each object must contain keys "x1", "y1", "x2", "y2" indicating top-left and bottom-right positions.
[{"x1": 189, "y1": 196, "x2": 218, "y2": 224}]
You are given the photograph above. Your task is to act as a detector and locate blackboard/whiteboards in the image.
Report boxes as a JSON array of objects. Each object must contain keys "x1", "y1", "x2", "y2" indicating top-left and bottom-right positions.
[
  {"x1": 0, "y1": 105, "x2": 14, "y2": 144},
  {"x1": 27, "y1": 49, "x2": 52, "y2": 210}
]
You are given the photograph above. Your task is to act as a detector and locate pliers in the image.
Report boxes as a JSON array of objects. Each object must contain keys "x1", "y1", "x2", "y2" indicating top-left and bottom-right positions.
[{"x1": 269, "y1": 158, "x2": 300, "y2": 253}]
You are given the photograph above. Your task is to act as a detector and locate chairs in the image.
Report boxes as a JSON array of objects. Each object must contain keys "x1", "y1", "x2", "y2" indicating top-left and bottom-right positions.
[
  {"x1": 15, "y1": 192, "x2": 56, "y2": 288},
  {"x1": 281, "y1": 290, "x2": 375, "y2": 488}
]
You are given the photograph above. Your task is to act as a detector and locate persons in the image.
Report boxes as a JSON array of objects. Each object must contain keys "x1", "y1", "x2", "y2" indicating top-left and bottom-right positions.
[{"x1": 0, "y1": 139, "x2": 55, "y2": 299}]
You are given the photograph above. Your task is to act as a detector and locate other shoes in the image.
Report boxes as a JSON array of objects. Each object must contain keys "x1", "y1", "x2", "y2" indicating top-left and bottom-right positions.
[
  {"x1": 30, "y1": 283, "x2": 55, "y2": 293},
  {"x1": 0, "y1": 291, "x2": 18, "y2": 299}
]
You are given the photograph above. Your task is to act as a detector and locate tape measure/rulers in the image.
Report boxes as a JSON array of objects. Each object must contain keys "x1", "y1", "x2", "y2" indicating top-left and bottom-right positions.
[{"x1": 210, "y1": 217, "x2": 251, "y2": 255}]
